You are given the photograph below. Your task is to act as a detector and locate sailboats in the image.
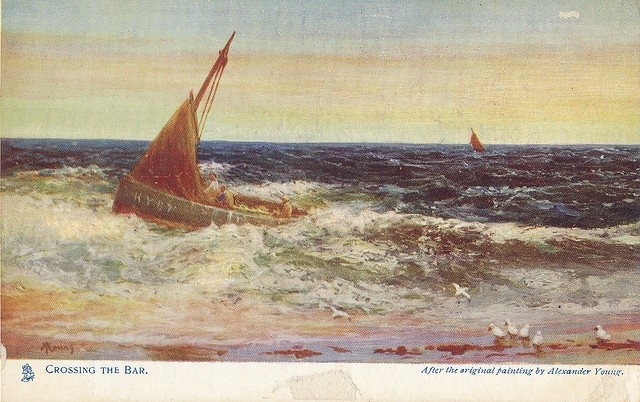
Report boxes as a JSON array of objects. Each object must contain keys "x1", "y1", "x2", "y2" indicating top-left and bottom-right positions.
[
  {"x1": 468, "y1": 127, "x2": 485, "y2": 151},
  {"x1": 115, "y1": 31, "x2": 311, "y2": 234}
]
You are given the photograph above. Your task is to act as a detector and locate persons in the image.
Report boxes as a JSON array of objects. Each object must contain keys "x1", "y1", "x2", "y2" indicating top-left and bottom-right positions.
[
  {"x1": 280, "y1": 192, "x2": 296, "y2": 220},
  {"x1": 204, "y1": 172, "x2": 220, "y2": 193},
  {"x1": 222, "y1": 183, "x2": 235, "y2": 209}
]
